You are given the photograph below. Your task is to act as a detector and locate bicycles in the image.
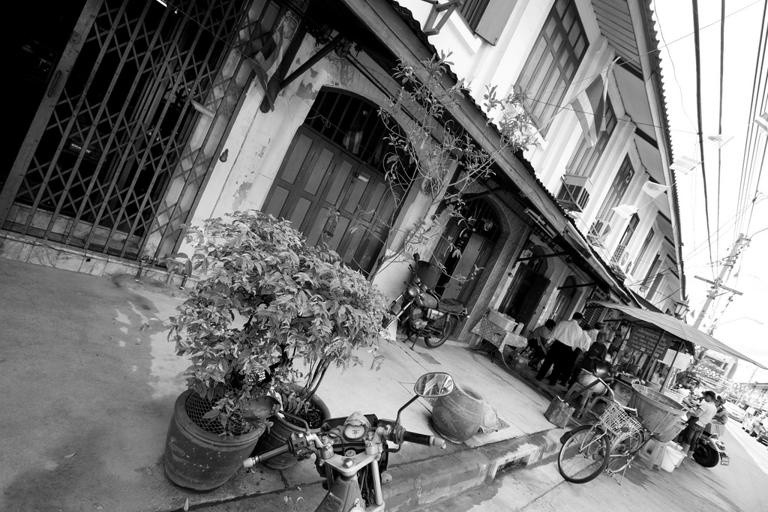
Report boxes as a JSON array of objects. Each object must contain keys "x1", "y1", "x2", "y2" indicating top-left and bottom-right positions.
[{"x1": 556, "y1": 375, "x2": 656, "y2": 487}]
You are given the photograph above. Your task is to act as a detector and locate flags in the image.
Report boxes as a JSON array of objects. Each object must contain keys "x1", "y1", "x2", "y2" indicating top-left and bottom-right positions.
[
  {"x1": 612, "y1": 112, "x2": 768, "y2": 220},
  {"x1": 570, "y1": 59, "x2": 607, "y2": 148}
]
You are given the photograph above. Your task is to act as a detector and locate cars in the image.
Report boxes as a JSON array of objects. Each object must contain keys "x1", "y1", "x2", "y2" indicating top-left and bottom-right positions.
[{"x1": 726, "y1": 402, "x2": 768, "y2": 448}]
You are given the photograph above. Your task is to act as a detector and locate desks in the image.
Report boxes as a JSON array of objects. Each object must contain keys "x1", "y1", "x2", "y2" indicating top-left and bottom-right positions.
[{"x1": 471, "y1": 317, "x2": 527, "y2": 365}]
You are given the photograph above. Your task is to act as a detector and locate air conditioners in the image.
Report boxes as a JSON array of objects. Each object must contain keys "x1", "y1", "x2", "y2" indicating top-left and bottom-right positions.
[{"x1": 592, "y1": 218, "x2": 632, "y2": 276}]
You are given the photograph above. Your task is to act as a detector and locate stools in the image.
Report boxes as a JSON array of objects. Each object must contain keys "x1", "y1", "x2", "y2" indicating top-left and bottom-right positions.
[{"x1": 563, "y1": 381, "x2": 591, "y2": 419}]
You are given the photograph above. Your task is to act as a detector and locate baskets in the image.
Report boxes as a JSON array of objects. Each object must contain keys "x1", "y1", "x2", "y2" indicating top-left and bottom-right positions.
[{"x1": 629, "y1": 378, "x2": 690, "y2": 444}]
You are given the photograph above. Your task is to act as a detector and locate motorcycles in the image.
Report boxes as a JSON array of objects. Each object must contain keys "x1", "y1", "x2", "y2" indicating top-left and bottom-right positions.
[
  {"x1": 241, "y1": 370, "x2": 454, "y2": 511},
  {"x1": 678, "y1": 388, "x2": 730, "y2": 468},
  {"x1": 375, "y1": 251, "x2": 469, "y2": 351}
]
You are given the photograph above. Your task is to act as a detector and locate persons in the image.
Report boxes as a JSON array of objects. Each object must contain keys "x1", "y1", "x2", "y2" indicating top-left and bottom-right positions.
[
  {"x1": 709, "y1": 393, "x2": 728, "y2": 424},
  {"x1": 534, "y1": 311, "x2": 632, "y2": 387},
  {"x1": 525, "y1": 318, "x2": 556, "y2": 372},
  {"x1": 682, "y1": 390, "x2": 717, "y2": 462}
]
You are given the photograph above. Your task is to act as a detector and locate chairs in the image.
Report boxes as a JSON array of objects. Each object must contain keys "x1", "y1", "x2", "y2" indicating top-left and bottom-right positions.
[{"x1": 518, "y1": 330, "x2": 538, "y2": 360}]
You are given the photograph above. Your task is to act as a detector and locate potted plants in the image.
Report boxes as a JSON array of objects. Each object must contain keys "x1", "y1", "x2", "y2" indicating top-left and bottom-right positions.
[{"x1": 146, "y1": 202, "x2": 395, "y2": 493}]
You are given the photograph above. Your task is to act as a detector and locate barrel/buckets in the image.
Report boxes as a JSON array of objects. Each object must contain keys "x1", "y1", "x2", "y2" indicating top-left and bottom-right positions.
[{"x1": 661, "y1": 446, "x2": 683, "y2": 472}]
